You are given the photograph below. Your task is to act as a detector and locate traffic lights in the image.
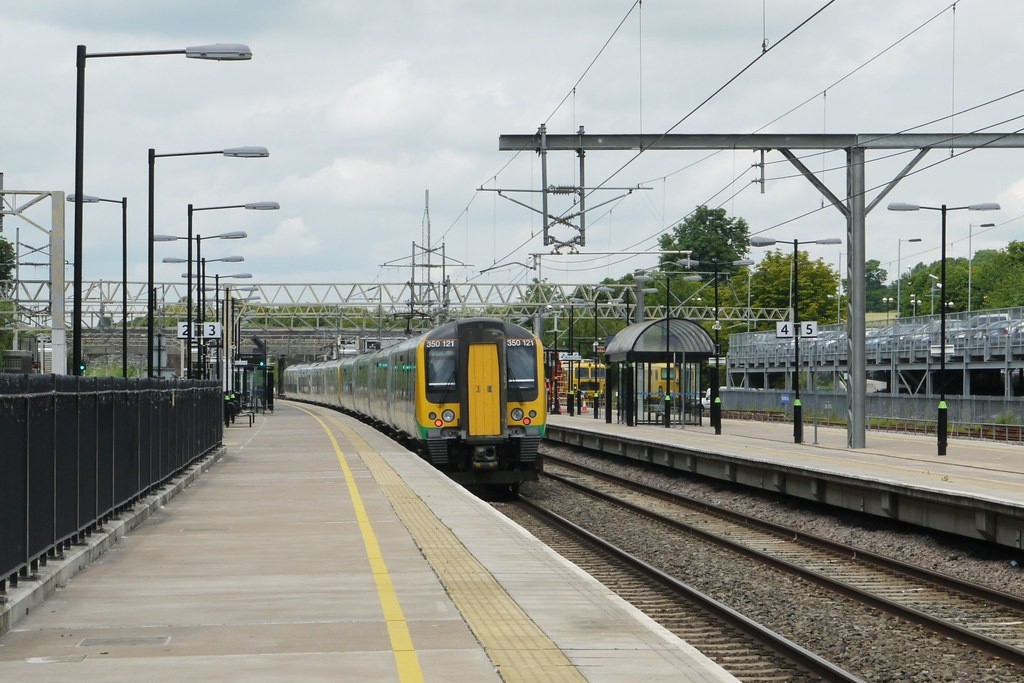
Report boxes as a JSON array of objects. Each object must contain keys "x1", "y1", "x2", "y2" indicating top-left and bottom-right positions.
[
  {"x1": 259, "y1": 361, "x2": 265, "y2": 366},
  {"x1": 80, "y1": 361, "x2": 86, "y2": 370}
]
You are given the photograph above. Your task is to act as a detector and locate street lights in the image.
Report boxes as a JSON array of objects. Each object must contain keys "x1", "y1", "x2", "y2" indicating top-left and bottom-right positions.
[
  {"x1": 72, "y1": 42, "x2": 253, "y2": 376},
  {"x1": 633, "y1": 276, "x2": 702, "y2": 429},
  {"x1": 882, "y1": 298, "x2": 893, "y2": 326},
  {"x1": 896, "y1": 239, "x2": 922, "y2": 319},
  {"x1": 146, "y1": 145, "x2": 270, "y2": 375},
  {"x1": 749, "y1": 235, "x2": 842, "y2": 443},
  {"x1": 680, "y1": 258, "x2": 755, "y2": 435},
  {"x1": 966, "y1": 223, "x2": 994, "y2": 312},
  {"x1": 64, "y1": 193, "x2": 128, "y2": 377},
  {"x1": 184, "y1": 201, "x2": 280, "y2": 379},
  {"x1": 885, "y1": 202, "x2": 1001, "y2": 456},
  {"x1": 597, "y1": 286, "x2": 660, "y2": 326},
  {"x1": 155, "y1": 231, "x2": 261, "y2": 380},
  {"x1": 569, "y1": 297, "x2": 624, "y2": 419}
]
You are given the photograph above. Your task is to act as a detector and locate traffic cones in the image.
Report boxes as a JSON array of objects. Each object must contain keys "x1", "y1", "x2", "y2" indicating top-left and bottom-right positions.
[{"x1": 580, "y1": 397, "x2": 588, "y2": 413}]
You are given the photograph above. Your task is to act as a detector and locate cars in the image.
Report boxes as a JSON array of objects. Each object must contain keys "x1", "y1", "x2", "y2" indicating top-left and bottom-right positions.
[
  {"x1": 774, "y1": 314, "x2": 1024, "y2": 353},
  {"x1": 701, "y1": 395, "x2": 710, "y2": 412}
]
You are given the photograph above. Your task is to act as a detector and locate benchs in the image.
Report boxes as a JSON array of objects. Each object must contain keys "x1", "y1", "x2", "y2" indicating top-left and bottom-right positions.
[{"x1": 651, "y1": 397, "x2": 689, "y2": 423}]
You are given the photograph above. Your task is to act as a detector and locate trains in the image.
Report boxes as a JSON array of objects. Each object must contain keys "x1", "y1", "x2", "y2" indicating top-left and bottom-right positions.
[
  {"x1": 283, "y1": 317, "x2": 548, "y2": 502},
  {"x1": 560, "y1": 359, "x2": 605, "y2": 408},
  {"x1": 649, "y1": 363, "x2": 680, "y2": 407}
]
